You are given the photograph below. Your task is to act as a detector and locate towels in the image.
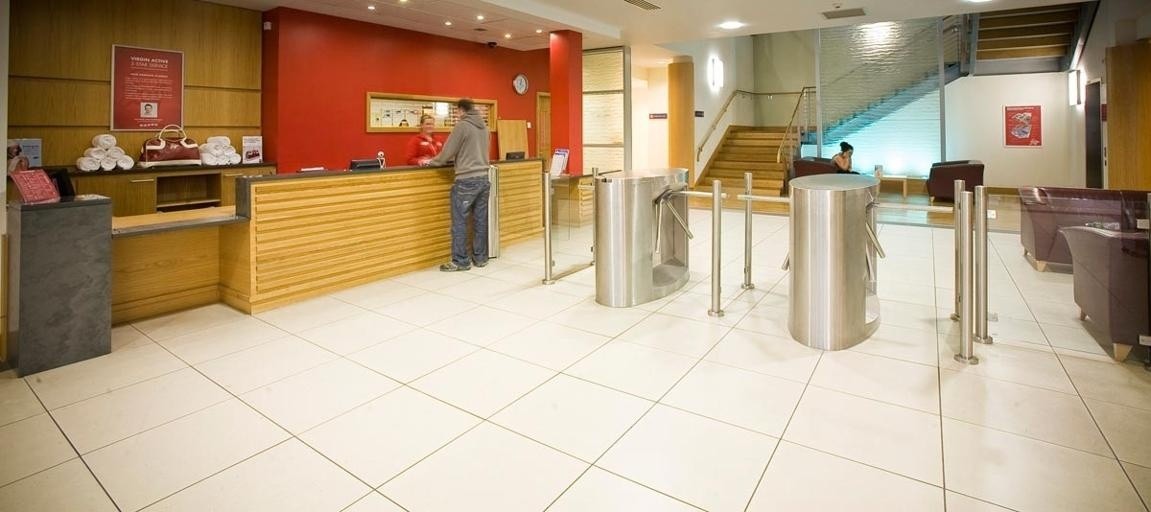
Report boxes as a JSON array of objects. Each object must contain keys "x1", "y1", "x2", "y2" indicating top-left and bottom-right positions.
[
  {"x1": 76, "y1": 133, "x2": 135, "y2": 172},
  {"x1": 199, "y1": 135, "x2": 242, "y2": 166}
]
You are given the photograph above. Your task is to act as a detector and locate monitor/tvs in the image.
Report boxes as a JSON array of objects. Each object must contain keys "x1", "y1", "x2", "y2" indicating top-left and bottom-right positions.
[
  {"x1": 351, "y1": 159, "x2": 384, "y2": 171},
  {"x1": 506, "y1": 152, "x2": 525, "y2": 160}
]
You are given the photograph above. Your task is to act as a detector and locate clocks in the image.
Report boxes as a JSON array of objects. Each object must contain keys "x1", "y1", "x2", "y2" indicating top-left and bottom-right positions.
[{"x1": 512, "y1": 73, "x2": 529, "y2": 96}]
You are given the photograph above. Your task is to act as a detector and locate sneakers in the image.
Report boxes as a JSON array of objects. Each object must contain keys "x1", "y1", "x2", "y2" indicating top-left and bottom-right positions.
[{"x1": 440, "y1": 261, "x2": 471, "y2": 271}]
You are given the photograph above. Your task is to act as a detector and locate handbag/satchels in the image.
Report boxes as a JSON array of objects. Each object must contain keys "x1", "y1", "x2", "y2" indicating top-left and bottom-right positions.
[{"x1": 136, "y1": 124, "x2": 202, "y2": 168}]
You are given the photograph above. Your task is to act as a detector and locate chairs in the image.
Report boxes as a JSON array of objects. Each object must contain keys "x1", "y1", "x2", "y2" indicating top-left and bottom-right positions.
[
  {"x1": 1018, "y1": 185, "x2": 1134, "y2": 275},
  {"x1": 791, "y1": 156, "x2": 841, "y2": 177},
  {"x1": 1059, "y1": 225, "x2": 1151, "y2": 363},
  {"x1": 926, "y1": 160, "x2": 985, "y2": 202},
  {"x1": 1120, "y1": 189, "x2": 1151, "y2": 229}
]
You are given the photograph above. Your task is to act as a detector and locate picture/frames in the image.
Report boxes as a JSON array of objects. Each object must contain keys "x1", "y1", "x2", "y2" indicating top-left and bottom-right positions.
[{"x1": 366, "y1": 90, "x2": 498, "y2": 134}]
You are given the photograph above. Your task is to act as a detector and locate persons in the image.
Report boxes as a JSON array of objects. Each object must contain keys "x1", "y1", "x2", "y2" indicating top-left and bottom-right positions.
[
  {"x1": 143, "y1": 104, "x2": 154, "y2": 116},
  {"x1": 7, "y1": 142, "x2": 29, "y2": 173},
  {"x1": 423, "y1": 99, "x2": 490, "y2": 272},
  {"x1": 406, "y1": 115, "x2": 444, "y2": 167},
  {"x1": 830, "y1": 142, "x2": 859, "y2": 174}
]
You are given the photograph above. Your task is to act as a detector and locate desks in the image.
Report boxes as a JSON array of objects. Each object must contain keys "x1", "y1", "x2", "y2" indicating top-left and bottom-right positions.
[{"x1": 880, "y1": 175, "x2": 909, "y2": 199}]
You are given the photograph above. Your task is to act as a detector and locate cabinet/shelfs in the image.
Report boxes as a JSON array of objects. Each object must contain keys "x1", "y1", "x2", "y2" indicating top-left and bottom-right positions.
[{"x1": 75, "y1": 161, "x2": 276, "y2": 217}]
